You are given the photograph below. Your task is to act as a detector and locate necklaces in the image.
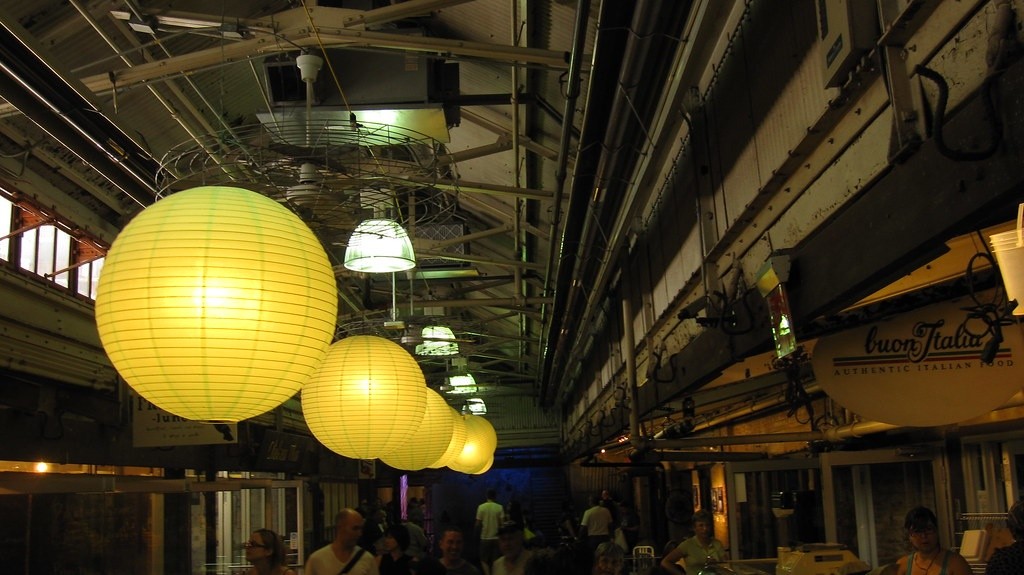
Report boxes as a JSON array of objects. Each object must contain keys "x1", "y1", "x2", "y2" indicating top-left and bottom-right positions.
[{"x1": 915, "y1": 548, "x2": 941, "y2": 575}]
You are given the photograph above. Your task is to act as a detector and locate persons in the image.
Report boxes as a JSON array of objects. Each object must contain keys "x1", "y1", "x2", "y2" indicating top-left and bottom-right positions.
[
  {"x1": 895, "y1": 506, "x2": 973, "y2": 575},
  {"x1": 475, "y1": 489, "x2": 504, "y2": 575},
  {"x1": 244, "y1": 497, "x2": 490, "y2": 575},
  {"x1": 985, "y1": 500, "x2": 1024, "y2": 575},
  {"x1": 493, "y1": 490, "x2": 729, "y2": 575}
]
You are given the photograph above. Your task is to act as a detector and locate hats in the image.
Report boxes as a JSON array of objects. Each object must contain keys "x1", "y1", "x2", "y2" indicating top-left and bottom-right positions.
[
  {"x1": 494, "y1": 520, "x2": 524, "y2": 537},
  {"x1": 904, "y1": 506, "x2": 936, "y2": 530}
]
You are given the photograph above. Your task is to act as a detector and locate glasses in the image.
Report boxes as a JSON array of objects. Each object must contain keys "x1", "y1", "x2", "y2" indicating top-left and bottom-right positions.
[
  {"x1": 910, "y1": 526, "x2": 936, "y2": 537},
  {"x1": 244, "y1": 541, "x2": 269, "y2": 550}
]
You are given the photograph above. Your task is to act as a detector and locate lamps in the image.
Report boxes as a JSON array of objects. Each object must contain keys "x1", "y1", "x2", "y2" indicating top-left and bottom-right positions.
[{"x1": 94, "y1": 185, "x2": 497, "y2": 475}]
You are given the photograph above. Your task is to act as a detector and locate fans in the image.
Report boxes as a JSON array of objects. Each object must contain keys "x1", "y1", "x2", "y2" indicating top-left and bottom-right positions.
[
  {"x1": 335, "y1": 260, "x2": 483, "y2": 359},
  {"x1": 154, "y1": 46, "x2": 459, "y2": 248}
]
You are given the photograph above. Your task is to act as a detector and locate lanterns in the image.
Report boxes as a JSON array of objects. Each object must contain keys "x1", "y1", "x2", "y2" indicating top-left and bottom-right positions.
[{"x1": 94, "y1": 186, "x2": 497, "y2": 475}]
